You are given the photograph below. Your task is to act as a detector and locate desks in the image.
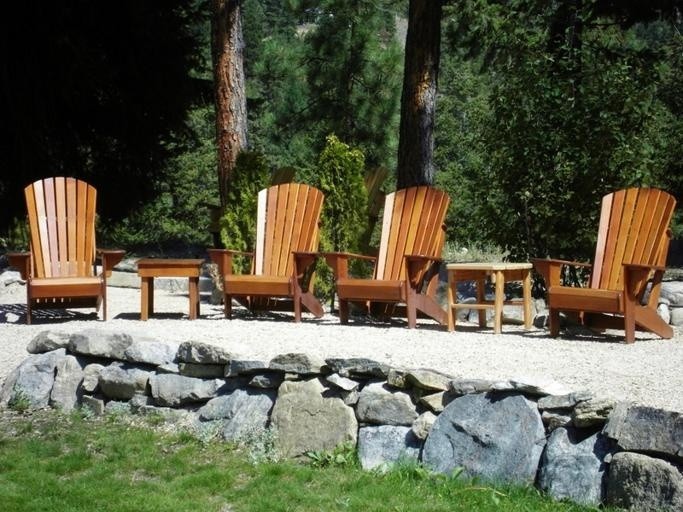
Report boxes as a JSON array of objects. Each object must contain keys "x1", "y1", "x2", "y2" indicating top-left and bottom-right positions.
[
  {"x1": 135, "y1": 257, "x2": 207, "y2": 321},
  {"x1": 445, "y1": 263, "x2": 534, "y2": 335}
]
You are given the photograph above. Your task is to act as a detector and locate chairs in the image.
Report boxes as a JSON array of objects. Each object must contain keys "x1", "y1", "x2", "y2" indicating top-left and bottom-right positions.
[
  {"x1": 320, "y1": 186, "x2": 451, "y2": 329},
  {"x1": 7, "y1": 177, "x2": 127, "y2": 325},
  {"x1": 208, "y1": 183, "x2": 325, "y2": 324},
  {"x1": 527, "y1": 187, "x2": 677, "y2": 344}
]
